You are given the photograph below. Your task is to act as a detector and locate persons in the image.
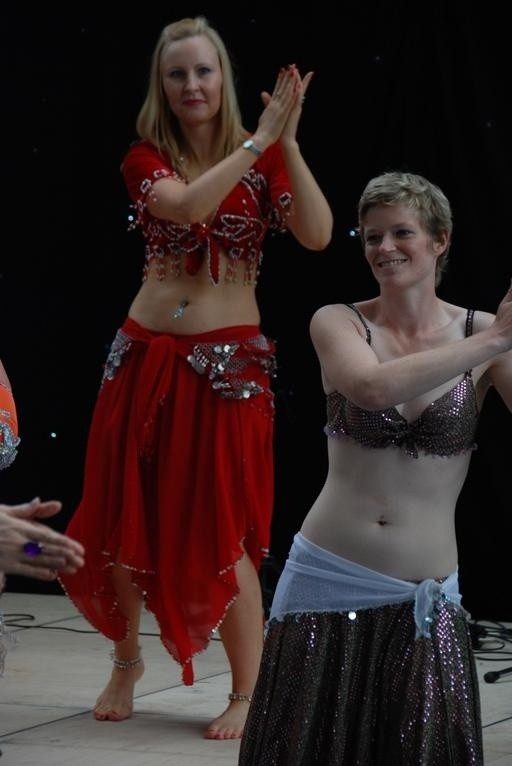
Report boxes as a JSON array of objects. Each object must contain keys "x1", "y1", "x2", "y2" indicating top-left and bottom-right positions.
[
  {"x1": 56, "y1": 14, "x2": 337, "y2": 746},
  {"x1": 0, "y1": 364, "x2": 87, "y2": 594},
  {"x1": 234, "y1": 167, "x2": 512, "y2": 766}
]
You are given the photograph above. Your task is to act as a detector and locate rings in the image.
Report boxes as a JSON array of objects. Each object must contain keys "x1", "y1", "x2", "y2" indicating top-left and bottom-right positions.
[{"x1": 22, "y1": 539, "x2": 42, "y2": 558}]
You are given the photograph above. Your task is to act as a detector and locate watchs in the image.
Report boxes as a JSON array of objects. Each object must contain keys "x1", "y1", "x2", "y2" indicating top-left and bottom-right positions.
[{"x1": 242, "y1": 138, "x2": 264, "y2": 156}]
